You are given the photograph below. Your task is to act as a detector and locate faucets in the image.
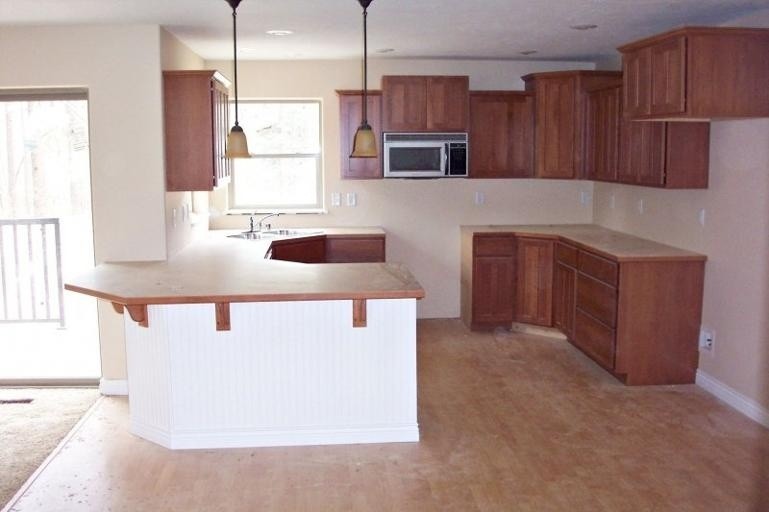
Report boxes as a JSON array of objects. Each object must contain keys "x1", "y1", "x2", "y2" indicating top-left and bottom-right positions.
[{"x1": 249, "y1": 212, "x2": 280, "y2": 231}]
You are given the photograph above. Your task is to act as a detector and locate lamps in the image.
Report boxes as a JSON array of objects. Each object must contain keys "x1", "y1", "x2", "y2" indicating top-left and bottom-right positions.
[
  {"x1": 348, "y1": 0, "x2": 379, "y2": 163},
  {"x1": 226, "y1": 0, "x2": 251, "y2": 161}
]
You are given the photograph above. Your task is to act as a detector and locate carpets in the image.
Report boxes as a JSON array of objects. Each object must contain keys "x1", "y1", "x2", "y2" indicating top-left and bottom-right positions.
[{"x1": 1, "y1": 382, "x2": 103, "y2": 512}]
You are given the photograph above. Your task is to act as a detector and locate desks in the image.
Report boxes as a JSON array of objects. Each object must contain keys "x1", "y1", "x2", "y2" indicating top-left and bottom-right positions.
[{"x1": 61, "y1": 259, "x2": 425, "y2": 447}]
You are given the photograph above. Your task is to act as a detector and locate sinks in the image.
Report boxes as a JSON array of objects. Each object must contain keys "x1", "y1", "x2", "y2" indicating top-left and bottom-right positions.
[
  {"x1": 226, "y1": 233, "x2": 275, "y2": 240},
  {"x1": 261, "y1": 228, "x2": 325, "y2": 236}
]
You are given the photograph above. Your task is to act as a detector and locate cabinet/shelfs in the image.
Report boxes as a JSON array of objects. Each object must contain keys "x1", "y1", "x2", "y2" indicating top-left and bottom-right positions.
[
  {"x1": 335, "y1": 88, "x2": 381, "y2": 180},
  {"x1": 553, "y1": 239, "x2": 574, "y2": 337},
  {"x1": 470, "y1": 88, "x2": 530, "y2": 179},
  {"x1": 574, "y1": 249, "x2": 703, "y2": 388},
  {"x1": 515, "y1": 234, "x2": 556, "y2": 326},
  {"x1": 162, "y1": 68, "x2": 234, "y2": 193},
  {"x1": 274, "y1": 236, "x2": 328, "y2": 265},
  {"x1": 613, "y1": 23, "x2": 768, "y2": 124},
  {"x1": 328, "y1": 236, "x2": 387, "y2": 264},
  {"x1": 530, "y1": 69, "x2": 583, "y2": 179},
  {"x1": 458, "y1": 225, "x2": 515, "y2": 332},
  {"x1": 581, "y1": 78, "x2": 708, "y2": 187},
  {"x1": 383, "y1": 73, "x2": 469, "y2": 133}
]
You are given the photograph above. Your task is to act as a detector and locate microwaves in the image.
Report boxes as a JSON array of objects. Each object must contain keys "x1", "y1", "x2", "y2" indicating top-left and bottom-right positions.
[{"x1": 382, "y1": 132, "x2": 469, "y2": 178}]
